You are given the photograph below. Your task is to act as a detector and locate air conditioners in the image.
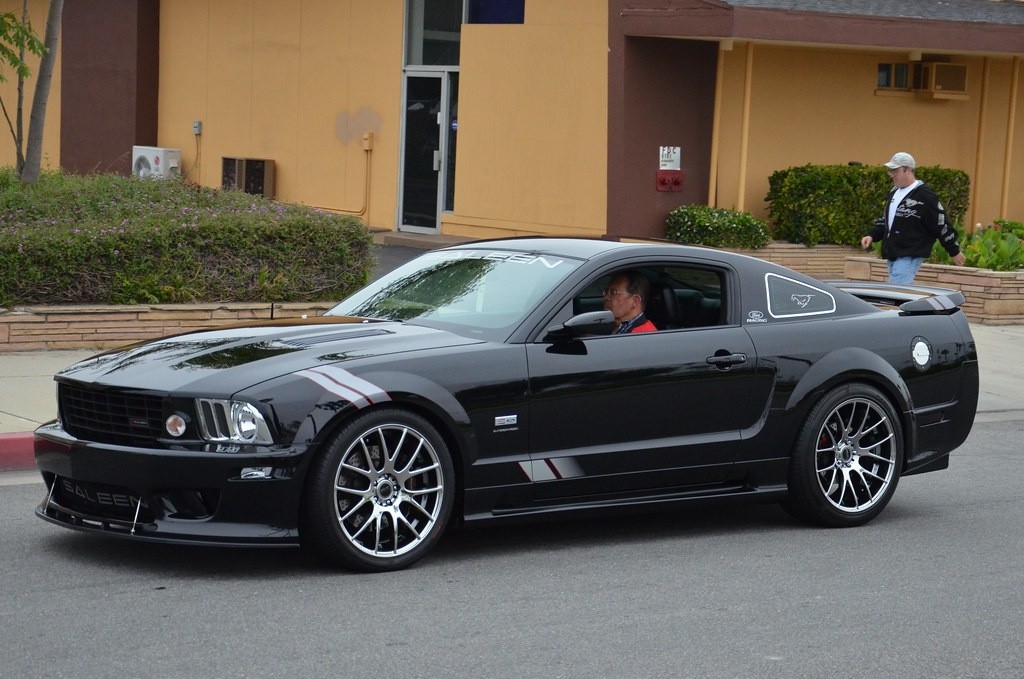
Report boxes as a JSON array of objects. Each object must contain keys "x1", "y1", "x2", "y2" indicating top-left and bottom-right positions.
[{"x1": 132, "y1": 146, "x2": 181, "y2": 179}]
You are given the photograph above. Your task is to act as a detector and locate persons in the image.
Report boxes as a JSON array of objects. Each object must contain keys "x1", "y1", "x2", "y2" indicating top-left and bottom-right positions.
[
  {"x1": 603, "y1": 268, "x2": 660, "y2": 335},
  {"x1": 860, "y1": 151, "x2": 967, "y2": 286}
]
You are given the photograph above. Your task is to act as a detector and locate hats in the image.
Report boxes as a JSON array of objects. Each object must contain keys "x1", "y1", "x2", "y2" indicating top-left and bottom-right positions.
[{"x1": 884, "y1": 152, "x2": 916, "y2": 169}]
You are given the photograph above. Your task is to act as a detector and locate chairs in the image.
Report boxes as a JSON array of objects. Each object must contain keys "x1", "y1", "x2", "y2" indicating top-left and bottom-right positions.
[{"x1": 646, "y1": 282, "x2": 723, "y2": 333}]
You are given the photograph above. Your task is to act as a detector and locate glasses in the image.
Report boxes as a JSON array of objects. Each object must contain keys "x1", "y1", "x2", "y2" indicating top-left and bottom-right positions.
[{"x1": 602, "y1": 287, "x2": 632, "y2": 296}]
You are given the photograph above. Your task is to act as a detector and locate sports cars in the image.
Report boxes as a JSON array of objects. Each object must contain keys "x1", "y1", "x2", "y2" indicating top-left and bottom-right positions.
[{"x1": 31, "y1": 237, "x2": 980, "y2": 575}]
